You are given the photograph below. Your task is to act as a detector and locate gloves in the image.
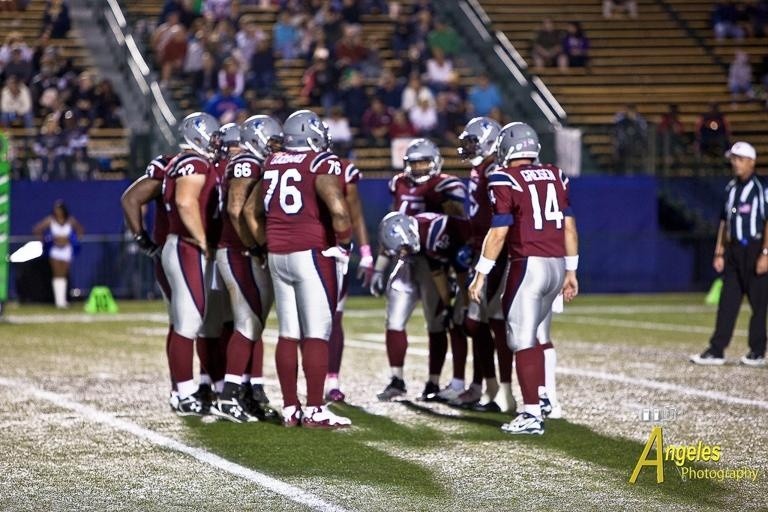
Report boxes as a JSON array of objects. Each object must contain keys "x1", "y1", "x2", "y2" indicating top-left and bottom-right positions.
[
  {"x1": 240, "y1": 243, "x2": 271, "y2": 275},
  {"x1": 320, "y1": 243, "x2": 390, "y2": 299},
  {"x1": 130, "y1": 230, "x2": 162, "y2": 264}
]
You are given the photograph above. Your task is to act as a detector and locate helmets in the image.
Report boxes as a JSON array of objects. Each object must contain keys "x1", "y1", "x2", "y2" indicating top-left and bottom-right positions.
[
  {"x1": 402, "y1": 117, "x2": 541, "y2": 184},
  {"x1": 175, "y1": 110, "x2": 333, "y2": 153},
  {"x1": 377, "y1": 212, "x2": 422, "y2": 261}
]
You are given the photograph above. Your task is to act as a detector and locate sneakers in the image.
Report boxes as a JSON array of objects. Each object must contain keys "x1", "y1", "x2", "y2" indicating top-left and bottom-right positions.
[
  {"x1": 691, "y1": 346, "x2": 724, "y2": 365},
  {"x1": 741, "y1": 348, "x2": 767, "y2": 364},
  {"x1": 168, "y1": 381, "x2": 352, "y2": 429},
  {"x1": 376, "y1": 378, "x2": 550, "y2": 436}
]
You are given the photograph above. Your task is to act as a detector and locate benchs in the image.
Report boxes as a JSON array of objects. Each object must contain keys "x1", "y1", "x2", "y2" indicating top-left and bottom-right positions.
[{"x1": 0, "y1": 0, "x2": 767, "y2": 180}]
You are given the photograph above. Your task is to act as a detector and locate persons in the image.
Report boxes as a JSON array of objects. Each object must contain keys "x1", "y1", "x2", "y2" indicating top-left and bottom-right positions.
[
  {"x1": 467, "y1": 118, "x2": 579, "y2": 439},
  {"x1": 2, "y1": 1, "x2": 768, "y2": 427}
]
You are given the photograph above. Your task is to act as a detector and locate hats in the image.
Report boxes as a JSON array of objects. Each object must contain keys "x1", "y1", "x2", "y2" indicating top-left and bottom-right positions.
[{"x1": 726, "y1": 141, "x2": 755, "y2": 159}]
